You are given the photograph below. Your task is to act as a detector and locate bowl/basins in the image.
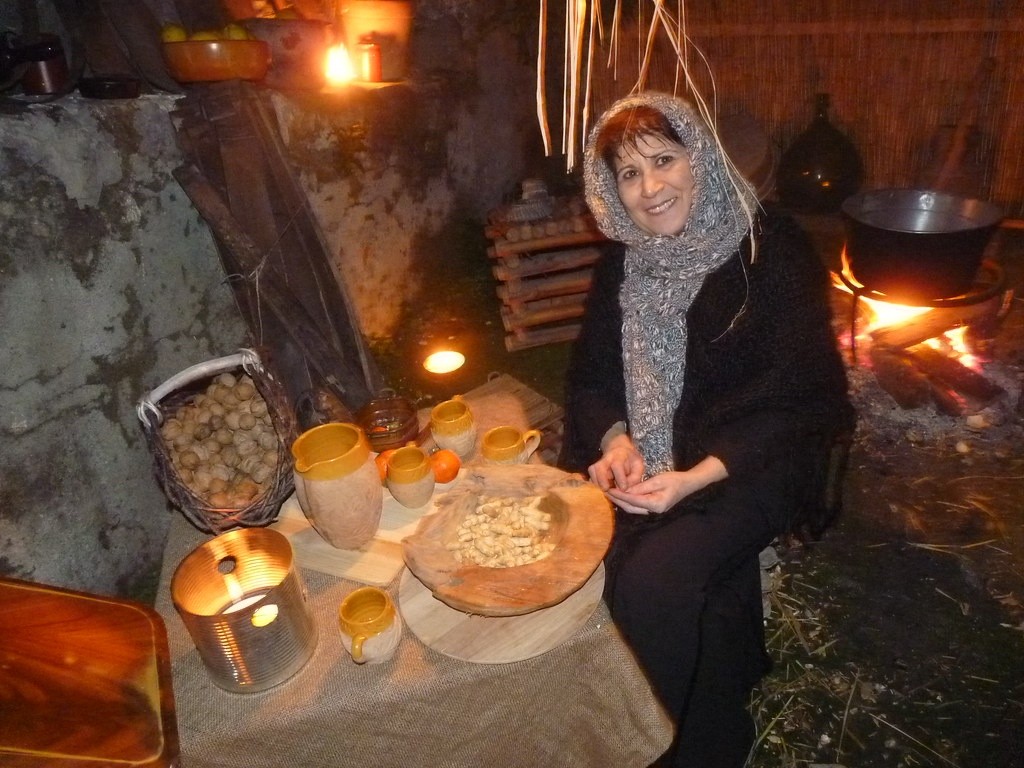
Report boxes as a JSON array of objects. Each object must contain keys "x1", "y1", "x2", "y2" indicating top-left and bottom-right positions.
[
  {"x1": 161, "y1": 40, "x2": 271, "y2": 81},
  {"x1": 235, "y1": 17, "x2": 330, "y2": 63}
]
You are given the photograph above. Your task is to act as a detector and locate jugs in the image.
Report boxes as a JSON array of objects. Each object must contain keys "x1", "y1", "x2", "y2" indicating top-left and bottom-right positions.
[{"x1": 291, "y1": 423, "x2": 383, "y2": 550}]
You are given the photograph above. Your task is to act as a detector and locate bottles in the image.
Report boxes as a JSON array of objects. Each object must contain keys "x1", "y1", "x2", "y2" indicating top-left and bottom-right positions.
[{"x1": 776, "y1": 94, "x2": 862, "y2": 212}]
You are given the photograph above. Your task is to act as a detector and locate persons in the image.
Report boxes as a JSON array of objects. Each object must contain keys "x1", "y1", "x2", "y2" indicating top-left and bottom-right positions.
[{"x1": 556, "y1": 92, "x2": 850, "y2": 768}]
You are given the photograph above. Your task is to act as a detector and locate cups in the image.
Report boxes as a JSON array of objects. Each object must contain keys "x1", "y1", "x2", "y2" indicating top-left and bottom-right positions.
[
  {"x1": 354, "y1": 44, "x2": 382, "y2": 83},
  {"x1": 386, "y1": 441, "x2": 435, "y2": 509},
  {"x1": 337, "y1": 587, "x2": 402, "y2": 665},
  {"x1": 431, "y1": 395, "x2": 477, "y2": 457},
  {"x1": 482, "y1": 426, "x2": 541, "y2": 466}
]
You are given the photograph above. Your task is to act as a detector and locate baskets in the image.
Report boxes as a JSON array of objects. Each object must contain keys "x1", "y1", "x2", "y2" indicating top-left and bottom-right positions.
[{"x1": 137, "y1": 349, "x2": 297, "y2": 531}]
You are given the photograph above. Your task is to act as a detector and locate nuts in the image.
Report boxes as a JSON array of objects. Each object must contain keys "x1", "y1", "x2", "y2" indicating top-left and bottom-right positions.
[
  {"x1": 159, "y1": 373, "x2": 280, "y2": 511},
  {"x1": 457, "y1": 494, "x2": 555, "y2": 567}
]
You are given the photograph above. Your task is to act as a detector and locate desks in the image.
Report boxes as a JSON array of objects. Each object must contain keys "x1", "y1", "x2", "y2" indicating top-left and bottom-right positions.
[{"x1": 155, "y1": 392, "x2": 675, "y2": 768}]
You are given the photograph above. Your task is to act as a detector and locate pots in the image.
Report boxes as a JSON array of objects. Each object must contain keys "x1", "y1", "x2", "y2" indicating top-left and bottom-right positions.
[
  {"x1": 841, "y1": 189, "x2": 1002, "y2": 299},
  {"x1": 358, "y1": 388, "x2": 419, "y2": 453},
  {"x1": 21, "y1": 54, "x2": 68, "y2": 93}
]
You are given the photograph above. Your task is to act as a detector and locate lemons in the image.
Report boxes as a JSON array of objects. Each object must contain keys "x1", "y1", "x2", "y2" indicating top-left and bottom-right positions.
[{"x1": 160, "y1": 21, "x2": 257, "y2": 41}]
